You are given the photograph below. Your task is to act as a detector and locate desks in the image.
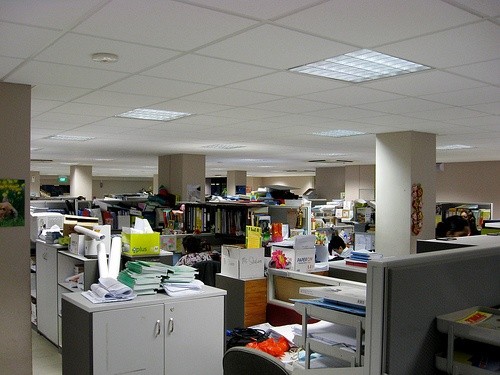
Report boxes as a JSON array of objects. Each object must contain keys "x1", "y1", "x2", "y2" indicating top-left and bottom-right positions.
[{"x1": 264, "y1": 232, "x2": 500, "y2": 374}]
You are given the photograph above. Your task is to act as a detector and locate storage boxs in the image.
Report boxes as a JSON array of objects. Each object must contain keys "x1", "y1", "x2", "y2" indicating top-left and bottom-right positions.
[
  {"x1": 271, "y1": 245, "x2": 316, "y2": 272},
  {"x1": 122, "y1": 233, "x2": 160, "y2": 257},
  {"x1": 221, "y1": 245, "x2": 265, "y2": 278}
]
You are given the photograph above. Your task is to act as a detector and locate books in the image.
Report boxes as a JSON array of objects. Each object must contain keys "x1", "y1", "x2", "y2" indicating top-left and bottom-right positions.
[
  {"x1": 163, "y1": 201, "x2": 271, "y2": 249},
  {"x1": 85, "y1": 256, "x2": 205, "y2": 299}
]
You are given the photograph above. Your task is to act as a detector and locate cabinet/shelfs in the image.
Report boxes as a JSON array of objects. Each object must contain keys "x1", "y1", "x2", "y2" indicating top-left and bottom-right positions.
[
  {"x1": 90, "y1": 291, "x2": 227, "y2": 375},
  {"x1": 436, "y1": 306, "x2": 500, "y2": 374},
  {"x1": 30, "y1": 241, "x2": 98, "y2": 349},
  {"x1": 289, "y1": 298, "x2": 366, "y2": 367},
  {"x1": 216, "y1": 272, "x2": 267, "y2": 329}
]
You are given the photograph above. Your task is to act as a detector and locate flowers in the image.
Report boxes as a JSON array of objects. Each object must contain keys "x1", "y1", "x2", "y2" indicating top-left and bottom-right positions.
[{"x1": 272, "y1": 250, "x2": 288, "y2": 269}]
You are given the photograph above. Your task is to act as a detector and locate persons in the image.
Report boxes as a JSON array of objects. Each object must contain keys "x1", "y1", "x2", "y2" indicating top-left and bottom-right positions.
[
  {"x1": 327, "y1": 235, "x2": 352, "y2": 257},
  {"x1": 436, "y1": 210, "x2": 480, "y2": 239},
  {"x1": 175, "y1": 235, "x2": 213, "y2": 267}
]
